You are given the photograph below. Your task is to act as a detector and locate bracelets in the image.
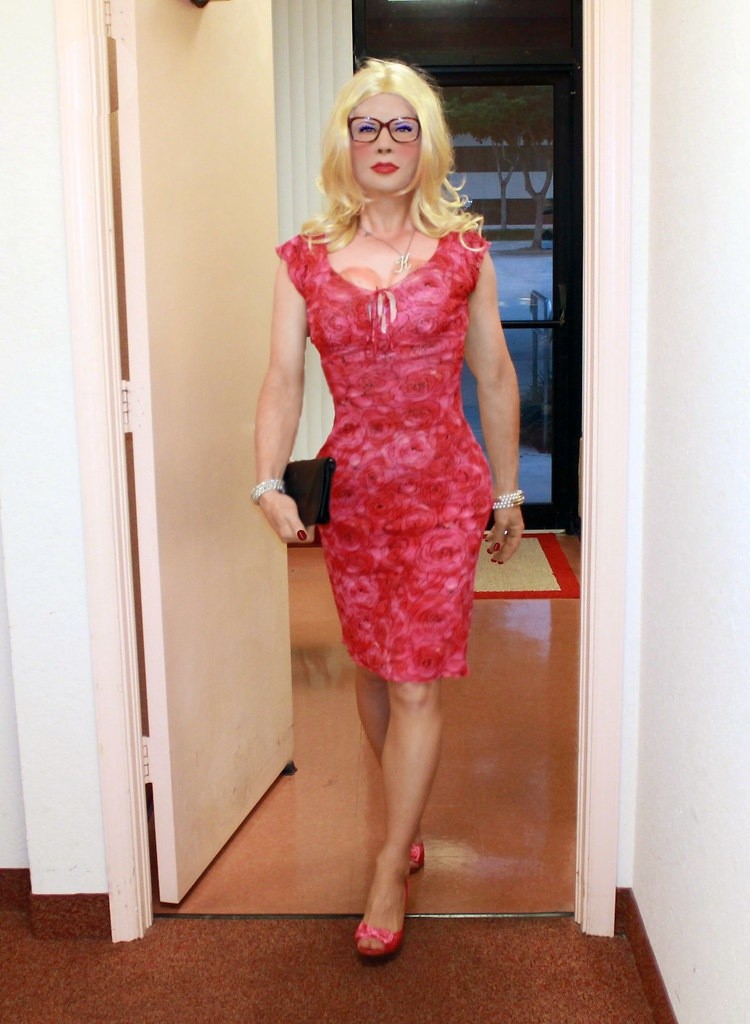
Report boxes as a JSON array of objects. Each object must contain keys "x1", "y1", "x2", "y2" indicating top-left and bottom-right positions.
[
  {"x1": 250, "y1": 479, "x2": 286, "y2": 505},
  {"x1": 493, "y1": 489, "x2": 525, "y2": 510}
]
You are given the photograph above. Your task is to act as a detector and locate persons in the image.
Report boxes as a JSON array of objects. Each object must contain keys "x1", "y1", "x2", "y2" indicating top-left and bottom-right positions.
[{"x1": 256, "y1": 60, "x2": 524, "y2": 957}]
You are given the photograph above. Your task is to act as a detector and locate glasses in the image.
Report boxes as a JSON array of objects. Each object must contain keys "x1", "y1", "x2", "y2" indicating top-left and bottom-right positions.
[{"x1": 347, "y1": 116, "x2": 420, "y2": 143}]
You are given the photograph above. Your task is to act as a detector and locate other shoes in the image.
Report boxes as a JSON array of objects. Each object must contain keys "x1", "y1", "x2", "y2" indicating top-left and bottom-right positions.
[
  {"x1": 408, "y1": 841, "x2": 425, "y2": 873},
  {"x1": 355, "y1": 877, "x2": 409, "y2": 957}
]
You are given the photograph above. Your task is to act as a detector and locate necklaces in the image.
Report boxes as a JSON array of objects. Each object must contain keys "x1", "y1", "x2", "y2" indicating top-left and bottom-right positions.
[{"x1": 360, "y1": 226, "x2": 417, "y2": 273}]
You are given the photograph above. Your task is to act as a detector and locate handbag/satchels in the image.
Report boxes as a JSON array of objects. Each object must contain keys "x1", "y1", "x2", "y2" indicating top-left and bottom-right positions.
[{"x1": 281, "y1": 457, "x2": 336, "y2": 527}]
